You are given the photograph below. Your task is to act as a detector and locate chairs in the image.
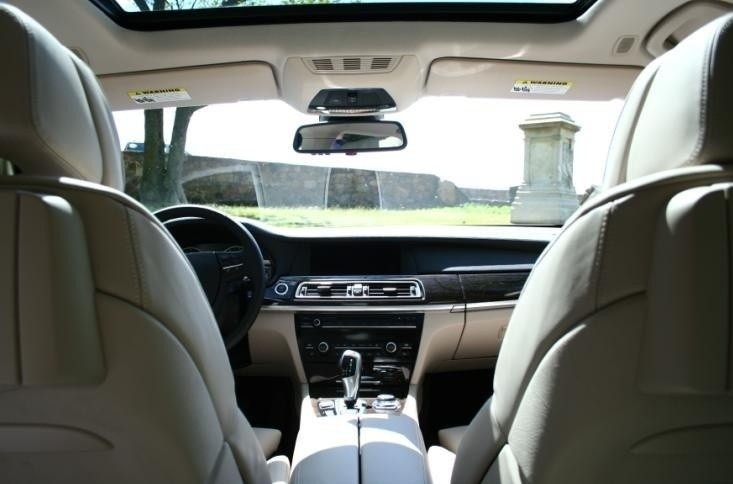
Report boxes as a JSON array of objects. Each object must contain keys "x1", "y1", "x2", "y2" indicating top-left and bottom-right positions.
[
  {"x1": 0, "y1": 2, "x2": 289, "y2": 483},
  {"x1": 428, "y1": 13, "x2": 732, "y2": 482}
]
